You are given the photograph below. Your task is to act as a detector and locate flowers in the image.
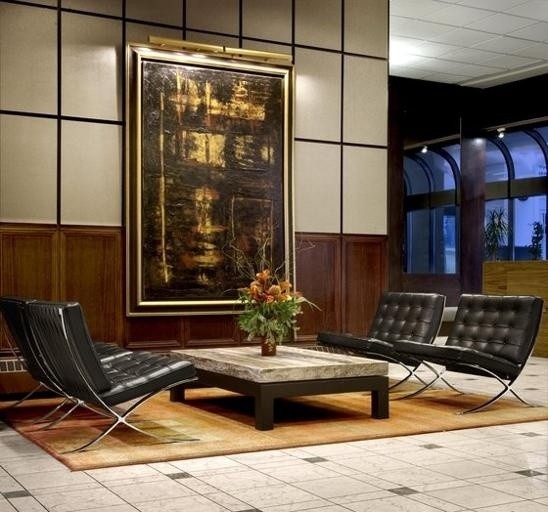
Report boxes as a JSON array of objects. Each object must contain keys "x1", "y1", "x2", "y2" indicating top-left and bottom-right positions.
[{"x1": 222, "y1": 269, "x2": 323, "y2": 342}]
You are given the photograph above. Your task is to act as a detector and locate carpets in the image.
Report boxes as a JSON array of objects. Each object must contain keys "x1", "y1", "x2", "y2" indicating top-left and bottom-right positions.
[{"x1": 0, "y1": 376, "x2": 548, "y2": 471}]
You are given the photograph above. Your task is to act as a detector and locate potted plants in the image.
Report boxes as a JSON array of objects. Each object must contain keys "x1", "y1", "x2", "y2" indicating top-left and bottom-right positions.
[
  {"x1": 528, "y1": 221, "x2": 545, "y2": 260},
  {"x1": 480, "y1": 207, "x2": 512, "y2": 261}
]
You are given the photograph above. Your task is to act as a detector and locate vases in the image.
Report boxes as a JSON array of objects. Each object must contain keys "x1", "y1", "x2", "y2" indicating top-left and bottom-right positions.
[{"x1": 261, "y1": 331, "x2": 277, "y2": 356}]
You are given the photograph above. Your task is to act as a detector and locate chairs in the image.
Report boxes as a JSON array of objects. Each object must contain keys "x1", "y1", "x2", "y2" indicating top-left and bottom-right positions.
[
  {"x1": 0, "y1": 296, "x2": 200, "y2": 455},
  {"x1": 316, "y1": 291, "x2": 543, "y2": 416}
]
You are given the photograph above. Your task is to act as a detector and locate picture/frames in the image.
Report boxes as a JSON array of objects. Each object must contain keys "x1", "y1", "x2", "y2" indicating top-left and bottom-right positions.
[{"x1": 124, "y1": 34, "x2": 296, "y2": 317}]
